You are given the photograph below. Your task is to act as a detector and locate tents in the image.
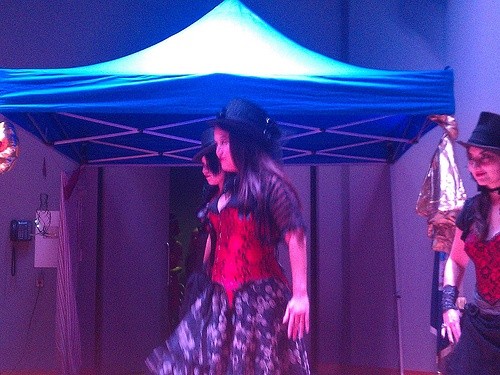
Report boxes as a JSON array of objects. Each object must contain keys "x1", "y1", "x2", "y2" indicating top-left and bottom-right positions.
[{"x1": 0, "y1": 0, "x2": 455, "y2": 375}]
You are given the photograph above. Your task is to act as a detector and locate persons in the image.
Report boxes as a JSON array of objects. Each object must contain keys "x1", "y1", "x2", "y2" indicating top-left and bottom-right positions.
[
  {"x1": 144, "y1": 96, "x2": 311, "y2": 375},
  {"x1": 178, "y1": 126, "x2": 231, "y2": 323},
  {"x1": 441, "y1": 111, "x2": 500, "y2": 375}
]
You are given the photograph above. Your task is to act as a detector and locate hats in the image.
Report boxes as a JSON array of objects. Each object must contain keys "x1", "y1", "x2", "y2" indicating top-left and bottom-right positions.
[
  {"x1": 458, "y1": 111, "x2": 500, "y2": 151},
  {"x1": 205, "y1": 98, "x2": 282, "y2": 143}
]
